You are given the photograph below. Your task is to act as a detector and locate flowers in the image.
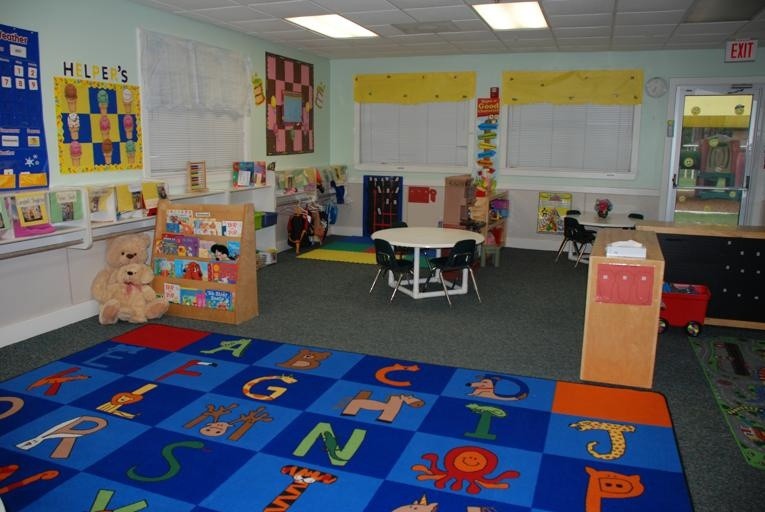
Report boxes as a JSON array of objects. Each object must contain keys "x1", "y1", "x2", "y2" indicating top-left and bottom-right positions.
[{"x1": 592, "y1": 197, "x2": 615, "y2": 213}]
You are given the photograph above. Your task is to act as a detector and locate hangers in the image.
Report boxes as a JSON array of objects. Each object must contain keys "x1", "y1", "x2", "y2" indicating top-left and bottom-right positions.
[{"x1": 281, "y1": 194, "x2": 338, "y2": 217}]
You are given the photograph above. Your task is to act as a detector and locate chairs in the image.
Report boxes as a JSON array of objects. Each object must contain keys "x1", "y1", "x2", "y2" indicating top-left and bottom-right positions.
[
  {"x1": 422, "y1": 239, "x2": 483, "y2": 307},
  {"x1": 385, "y1": 222, "x2": 417, "y2": 278},
  {"x1": 555, "y1": 216, "x2": 598, "y2": 271},
  {"x1": 622, "y1": 212, "x2": 645, "y2": 232},
  {"x1": 367, "y1": 237, "x2": 415, "y2": 303},
  {"x1": 564, "y1": 209, "x2": 588, "y2": 260}
]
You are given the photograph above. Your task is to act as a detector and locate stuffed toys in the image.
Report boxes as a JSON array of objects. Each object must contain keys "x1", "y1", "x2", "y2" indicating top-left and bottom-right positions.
[
  {"x1": 108, "y1": 263, "x2": 157, "y2": 324},
  {"x1": 88, "y1": 229, "x2": 168, "y2": 324}
]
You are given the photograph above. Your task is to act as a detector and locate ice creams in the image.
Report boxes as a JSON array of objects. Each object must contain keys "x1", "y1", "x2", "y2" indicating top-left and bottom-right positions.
[
  {"x1": 70, "y1": 142, "x2": 82, "y2": 167},
  {"x1": 103, "y1": 139, "x2": 112, "y2": 164},
  {"x1": 68, "y1": 113, "x2": 79, "y2": 139},
  {"x1": 126, "y1": 141, "x2": 136, "y2": 164},
  {"x1": 124, "y1": 116, "x2": 133, "y2": 139},
  {"x1": 97, "y1": 90, "x2": 108, "y2": 114},
  {"x1": 100, "y1": 116, "x2": 111, "y2": 139},
  {"x1": 122, "y1": 89, "x2": 134, "y2": 112},
  {"x1": 64, "y1": 84, "x2": 77, "y2": 113}
]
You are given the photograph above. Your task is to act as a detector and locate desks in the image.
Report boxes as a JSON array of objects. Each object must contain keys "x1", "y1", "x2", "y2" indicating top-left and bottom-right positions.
[
  {"x1": 66, "y1": 179, "x2": 169, "y2": 321},
  {"x1": 566, "y1": 213, "x2": 649, "y2": 266},
  {"x1": 169, "y1": 188, "x2": 224, "y2": 206},
  {"x1": 368, "y1": 226, "x2": 485, "y2": 299}
]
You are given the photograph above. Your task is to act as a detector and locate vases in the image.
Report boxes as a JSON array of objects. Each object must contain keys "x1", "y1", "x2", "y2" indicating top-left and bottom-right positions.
[{"x1": 599, "y1": 210, "x2": 608, "y2": 219}]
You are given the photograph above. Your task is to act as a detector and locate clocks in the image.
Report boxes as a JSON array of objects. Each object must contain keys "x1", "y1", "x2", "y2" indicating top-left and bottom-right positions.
[{"x1": 645, "y1": 77, "x2": 668, "y2": 99}]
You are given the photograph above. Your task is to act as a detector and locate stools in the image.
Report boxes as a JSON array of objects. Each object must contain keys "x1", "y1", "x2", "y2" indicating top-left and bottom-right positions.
[{"x1": 481, "y1": 245, "x2": 500, "y2": 267}]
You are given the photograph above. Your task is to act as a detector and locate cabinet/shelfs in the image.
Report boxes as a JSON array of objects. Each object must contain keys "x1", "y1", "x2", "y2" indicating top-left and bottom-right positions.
[
  {"x1": 362, "y1": 174, "x2": 403, "y2": 239},
  {"x1": 476, "y1": 190, "x2": 510, "y2": 246},
  {"x1": 633, "y1": 217, "x2": 765, "y2": 330},
  {"x1": 443, "y1": 176, "x2": 473, "y2": 226},
  {"x1": 579, "y1": 226, "x2": 666, "y2": 389},
  {"x1": 150, "y1": 200, "x2": 260, "y2": 323},
  {"x1": 205, "y1": 180, "x2": 279, "y2": 269},
  {"x1": 2, "y1": 184, "x2": 72, "y2": 350}
]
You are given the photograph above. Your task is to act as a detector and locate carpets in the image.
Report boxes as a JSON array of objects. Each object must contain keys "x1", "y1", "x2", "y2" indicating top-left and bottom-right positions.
[
  {"x1": 0, "y1": 323, "x2": 697, "y2": 512},
  {"x1": 688, "y1": 333, "x2": 765, "y2": 469}
]
